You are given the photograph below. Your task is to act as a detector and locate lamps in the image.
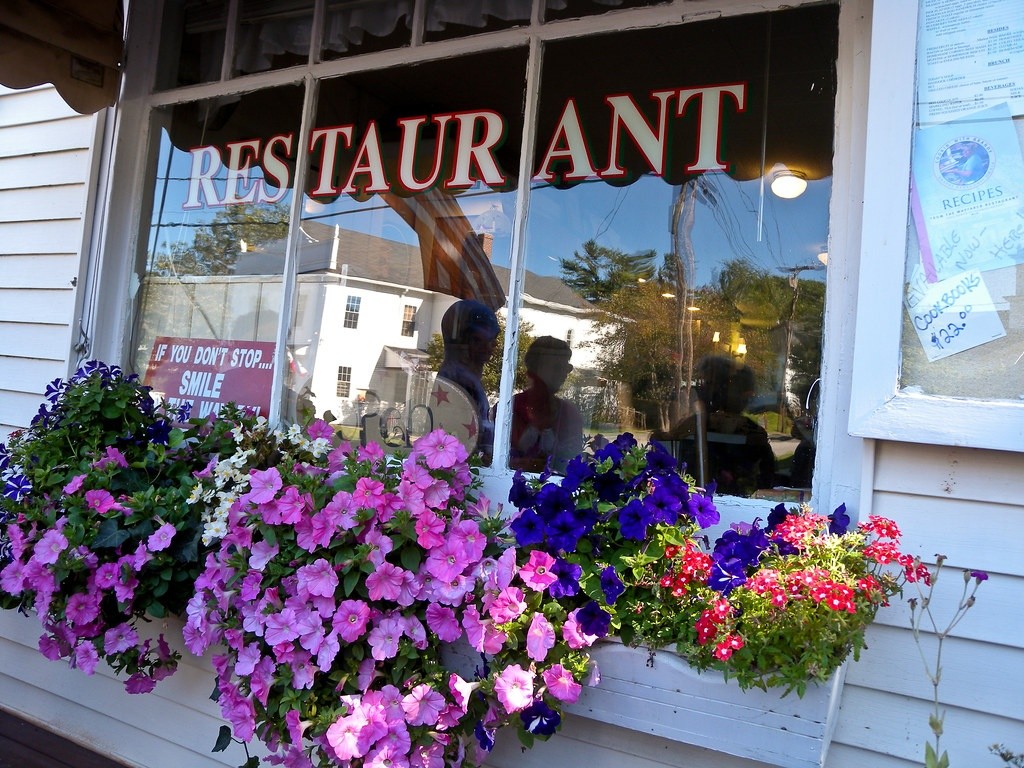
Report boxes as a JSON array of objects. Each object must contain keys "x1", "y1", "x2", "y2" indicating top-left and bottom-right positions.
[
  {"x1": 770, "y1": 162, "x2": 807, "y2": 199},
  {"x1": 732, "y1": 344, "x2": 748, "y2": 357}
]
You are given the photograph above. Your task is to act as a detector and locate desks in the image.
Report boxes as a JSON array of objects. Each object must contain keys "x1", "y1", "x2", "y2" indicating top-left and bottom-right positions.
[{"x1": 686, "y1": 432, "x2": 747, "y2": 474}]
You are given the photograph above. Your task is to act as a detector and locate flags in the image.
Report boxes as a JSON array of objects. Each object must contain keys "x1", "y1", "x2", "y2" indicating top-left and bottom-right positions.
[{"x1": 381, "y1": 186, "x2": 506, "y2": 313}]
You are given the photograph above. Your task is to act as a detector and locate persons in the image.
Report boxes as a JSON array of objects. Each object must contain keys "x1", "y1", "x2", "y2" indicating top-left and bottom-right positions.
[
  {"x1": 671, "y1": 355, "x2": 824, "y2": 498},
  {"x1": 425, "y1": 299, "x2": 584, "y2": 476}
]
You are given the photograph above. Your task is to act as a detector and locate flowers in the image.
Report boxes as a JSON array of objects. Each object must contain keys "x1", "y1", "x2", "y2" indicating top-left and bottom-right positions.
[{"x1": 0, "y1": 357, "x2": 935, "y2": 768}]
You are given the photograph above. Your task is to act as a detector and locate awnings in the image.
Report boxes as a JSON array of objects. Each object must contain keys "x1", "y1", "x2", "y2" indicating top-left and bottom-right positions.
[
  {"x1": 159, "y1": 0, "x2": 838, "y2": 204},
  {"x1": 0, "y1": 0, "x2": 124, "y2": 114}
]
